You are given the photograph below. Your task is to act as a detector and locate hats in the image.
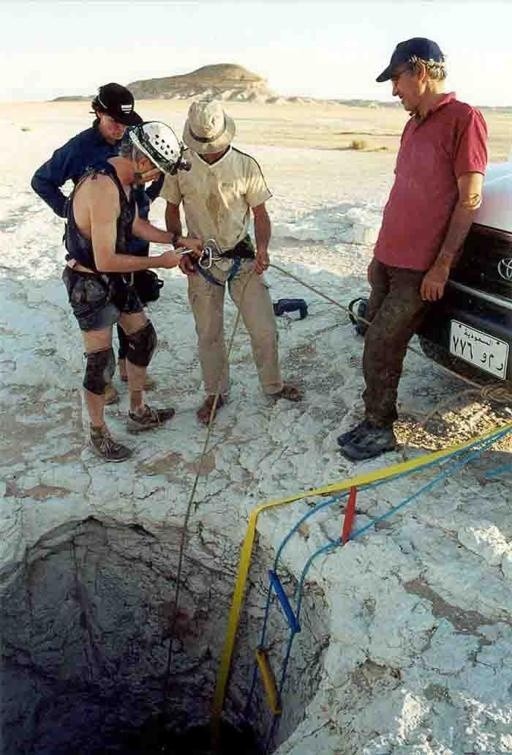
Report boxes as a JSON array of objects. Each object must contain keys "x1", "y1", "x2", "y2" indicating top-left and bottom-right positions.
[
  {"x1": 376, "y1": 37, "x2": 444, "y2": 82},
  {"x1": 182, "y1": 99, "x2": 236, "y2": 155},
  {"x1": 97, "y1": 82, "x2": 143, "y2": 127}
]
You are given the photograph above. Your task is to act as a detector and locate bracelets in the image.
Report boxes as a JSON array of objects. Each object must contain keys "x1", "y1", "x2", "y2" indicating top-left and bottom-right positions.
[{"x1": 172, "y1": 233, "x2": 179, "y2": 247}]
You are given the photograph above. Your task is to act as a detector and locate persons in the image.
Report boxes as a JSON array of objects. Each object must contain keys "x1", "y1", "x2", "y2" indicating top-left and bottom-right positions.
[
  {"x1": 62, "y1": 121, "x2": 205, "y2": 463},
  {"x1": 158, "y1": 96, "x2": 303, "y2": 424},
  {"x1": 31, "y1": 83, "x2": 165, "y2": 405},
  {"x1": 337, "y1": 38, "x2": 488, "y2": 462}
]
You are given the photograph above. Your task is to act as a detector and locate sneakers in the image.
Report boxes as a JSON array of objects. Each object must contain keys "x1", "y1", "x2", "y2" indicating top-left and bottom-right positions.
[
  {"x1": 337, "y1": 415, "x2": 396, "y2": 460},
  {"x1": 197, "y1": 394, "x2": 223, "y2": 424},
  {"x1": 277, "y1": 385, "x2": 302, "y2": 402},
  {"x1": 117, "y1": 358, "x2": 156, "y2": 388},
  {"x1": 105, "y1": 381, "x2": 119, "y2": 406},
  {"x1": 87, "y1": 432, "x2": 133, "y2": 462},
  {"x1": 126, "y1": 402, "x2": 175, "y2": 435}
]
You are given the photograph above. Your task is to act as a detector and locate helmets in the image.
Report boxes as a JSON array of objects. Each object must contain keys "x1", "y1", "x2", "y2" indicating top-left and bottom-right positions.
[{"x1": 129, "y1": 120, "x2": 192, "y2": 177}]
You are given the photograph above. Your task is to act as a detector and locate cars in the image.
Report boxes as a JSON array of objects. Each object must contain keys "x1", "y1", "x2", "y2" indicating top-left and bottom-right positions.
[{"x1": 399, "y1": 143, "x2": 511, "y2": 404}]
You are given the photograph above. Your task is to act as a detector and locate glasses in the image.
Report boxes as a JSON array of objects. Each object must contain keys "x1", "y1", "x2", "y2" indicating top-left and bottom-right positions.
[{"x1": 390, "y1": 66, "x2": 413, "y2": 86}]
